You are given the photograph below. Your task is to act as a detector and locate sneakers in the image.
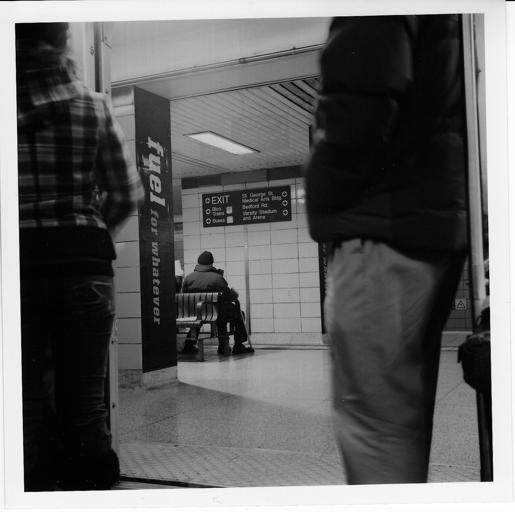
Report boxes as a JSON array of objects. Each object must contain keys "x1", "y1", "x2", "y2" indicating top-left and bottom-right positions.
[
  {"x1": 217, "y1": 344, "x2": 254, "y2": 354},
  {"x1": 183, "y1": 346, "x2": 198, "y2": 355}
]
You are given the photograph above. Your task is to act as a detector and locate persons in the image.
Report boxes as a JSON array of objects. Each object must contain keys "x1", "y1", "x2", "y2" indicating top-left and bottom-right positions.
[
  {"x1": 22, "y1": 24, "x2": 137, "y2": 490},
  {"x1": 304, "y1": 18, "x2": 467, "y2": 484},
  {"x1": 182, "y1": 247, "x2": 254, "y2": 354}
]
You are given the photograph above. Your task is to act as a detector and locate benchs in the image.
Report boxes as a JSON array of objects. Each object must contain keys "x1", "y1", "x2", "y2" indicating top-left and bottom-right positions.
[{"x1": 175, "y1": 292, "x2": 245, "y2": 362}]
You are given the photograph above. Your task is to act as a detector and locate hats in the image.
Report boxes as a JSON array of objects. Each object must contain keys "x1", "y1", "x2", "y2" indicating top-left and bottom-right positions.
[{"x1": 198, "y1": 251, "x2": 213, "y2": 265}]
